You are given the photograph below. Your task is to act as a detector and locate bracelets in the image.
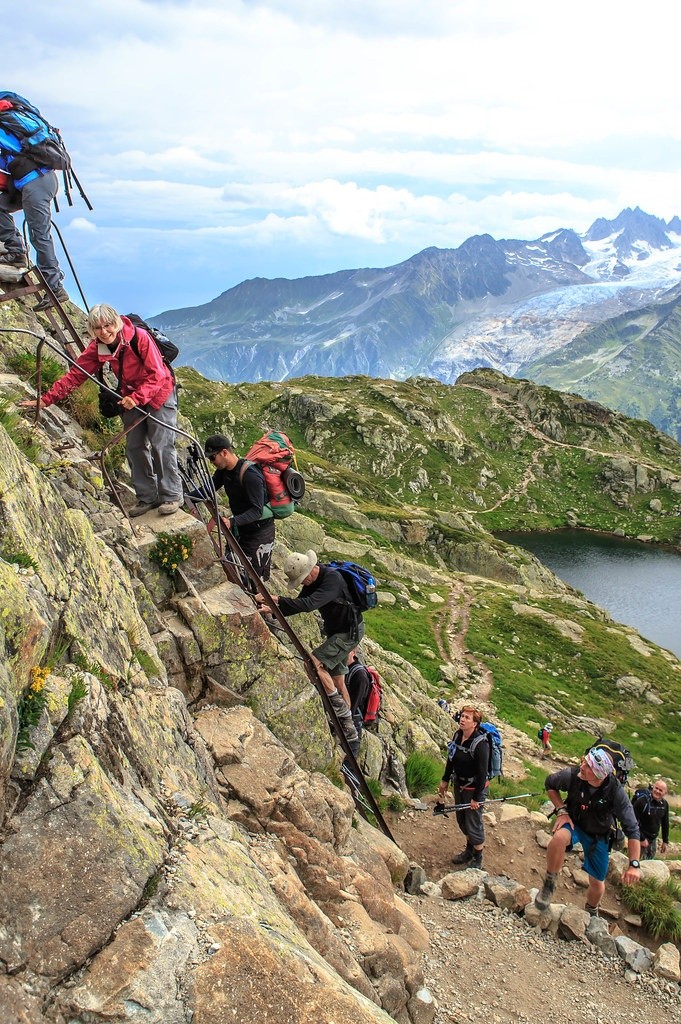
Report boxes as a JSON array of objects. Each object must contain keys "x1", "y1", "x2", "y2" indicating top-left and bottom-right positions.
[{"x1": 556, "y1": 806, "x2": 568, "y2": 816}]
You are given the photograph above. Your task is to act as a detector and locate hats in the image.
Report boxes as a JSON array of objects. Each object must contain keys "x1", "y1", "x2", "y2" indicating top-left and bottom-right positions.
[
  {"x1": 201, "y1": 434, "x2": 232, "y2": 458},
  {"x1": 284, "y1": 550, "x2": 317, "y2": 589},
  {"x1": 545, "y1": 723, "x2": 554, "y2": 729}
]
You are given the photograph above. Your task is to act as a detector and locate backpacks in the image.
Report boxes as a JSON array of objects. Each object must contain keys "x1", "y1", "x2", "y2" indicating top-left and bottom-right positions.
[
  {"x1": 631, "y1": 789, "x2": 665, "y2": 825},
  {"x1": 125, "y1": 313, "x2": 179, "y2": 364},
  {"x1": 585, "y1": 739, "x2": 630, "y2": 787},
  {"x1": 238, "y1": 429, "x2": 305, "y2": 520},
  {"x1": 345, "y1": 664, "x2": 383, "y2": 729},
  {"x1": 322, "y1": 560, "x2": 378, "y2": 612},
  {"x1": 0, "y1": 91, "x2": 71, "y2": 170},
  {"x1": 538, "y1": 729, "x2": 545, "y2": 741},
  {"x1": 448, "y1": 723, "x2": 503, "y2": 781}
]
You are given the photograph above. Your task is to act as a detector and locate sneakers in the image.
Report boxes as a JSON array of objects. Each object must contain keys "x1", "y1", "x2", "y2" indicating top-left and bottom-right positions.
[
  {"x1": 535, "y1": 875, "x2": 557, "y2": 910},
  {"x1": 32, "y1": 286, "x2": 69, "y2": 312},
  {"x1": 468, "y1": 857, "x2": 484, "y2": 869},
  {"x1": 452, "y1": 843, "x2": 475, "y2": 865},
  {"x1": 0, "y1": 251, "x2": 27, "y2": 268},
  {"x1": 584, "y1": 904, "x2": 598, "y2": 918}
]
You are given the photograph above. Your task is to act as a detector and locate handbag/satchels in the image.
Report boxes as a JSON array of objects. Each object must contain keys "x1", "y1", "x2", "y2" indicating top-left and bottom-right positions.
[{"x1": 98, "y1": 389, "x2": 125, "y2": 418}]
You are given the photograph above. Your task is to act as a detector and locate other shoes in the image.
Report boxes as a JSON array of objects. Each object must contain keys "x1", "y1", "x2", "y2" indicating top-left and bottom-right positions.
[
  {"x1": 158, "y1": 497, "x2": 184, "y2": 514},
  {"x1": 129, "y1": 497, "x2": 162, "y2": 517},
  {"x1": 541, "y1": 755, "x2": 547, "y2": 760},
  {"x1": 326, "y1": 700, "x2": 352, "y2": 718},
  {"x1": 332, "y1": 727, "x2": 359, "y2": 744},
  {"x1": 263, "y1": 615, "x2": 280, "y2": 627}
]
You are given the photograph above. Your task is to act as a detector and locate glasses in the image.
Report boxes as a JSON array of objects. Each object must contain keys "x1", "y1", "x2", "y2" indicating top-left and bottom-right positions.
[
  {"x1": 208, "y1": 451, "x2": 222, "y2": 462},
  {"x1": 589, "y1": 747, "x2": 610, "y2": 775}
]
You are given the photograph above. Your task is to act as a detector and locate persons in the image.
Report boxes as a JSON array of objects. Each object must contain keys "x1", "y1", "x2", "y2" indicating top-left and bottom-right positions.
[
  {"x1": 633, "y1": 781, "x2": 670, "y2": 860},
  {"x1": 535, "y1": 748, "x2": 641, "y2": 917},
  {"x1": 439, "y1": 706, "x2": 490, "y2": 869},
  {"x1": 453, "y1": 710, "x2": 462, "y2": 723},
  {"x1": 190, "y1": 434, "x2": 275, "y2": 624},
  {"x1": 344, "y1": 647, "x2": 369, "y2": 784},
  {"x1": 255, "y1": 551, "x2": 358, "y2": 741},
  {"x1": 21, "y1": 304, "x2": 184, "y2": 516},
  {"x1": 541, "y1": 723, "x2": 553, "y2": 760},
  {"x1": 0, "y1": 100, "x2": 69, "y2": 311},
  {"x1": 438, "y1": 699, "x2": 451, "y2": 714}
]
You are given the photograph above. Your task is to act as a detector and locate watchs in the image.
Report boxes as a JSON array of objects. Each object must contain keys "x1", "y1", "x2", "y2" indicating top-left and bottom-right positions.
[{"x1": 630, "y1": 860, "x2": 641, "y2": 868}]
